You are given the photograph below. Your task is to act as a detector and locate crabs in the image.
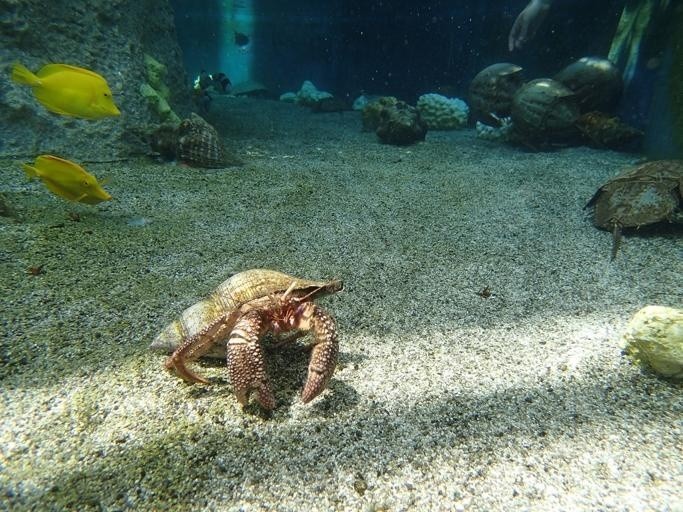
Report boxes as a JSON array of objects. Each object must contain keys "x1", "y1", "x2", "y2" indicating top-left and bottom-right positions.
[{"x1": 148, "y1": 268, "x2": 344, "y2": 412}]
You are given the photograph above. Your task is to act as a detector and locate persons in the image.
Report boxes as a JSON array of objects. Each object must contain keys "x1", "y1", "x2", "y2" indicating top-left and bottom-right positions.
[{"x1": 508, "y1": 0, "x2": 552, "y2": 52}]
